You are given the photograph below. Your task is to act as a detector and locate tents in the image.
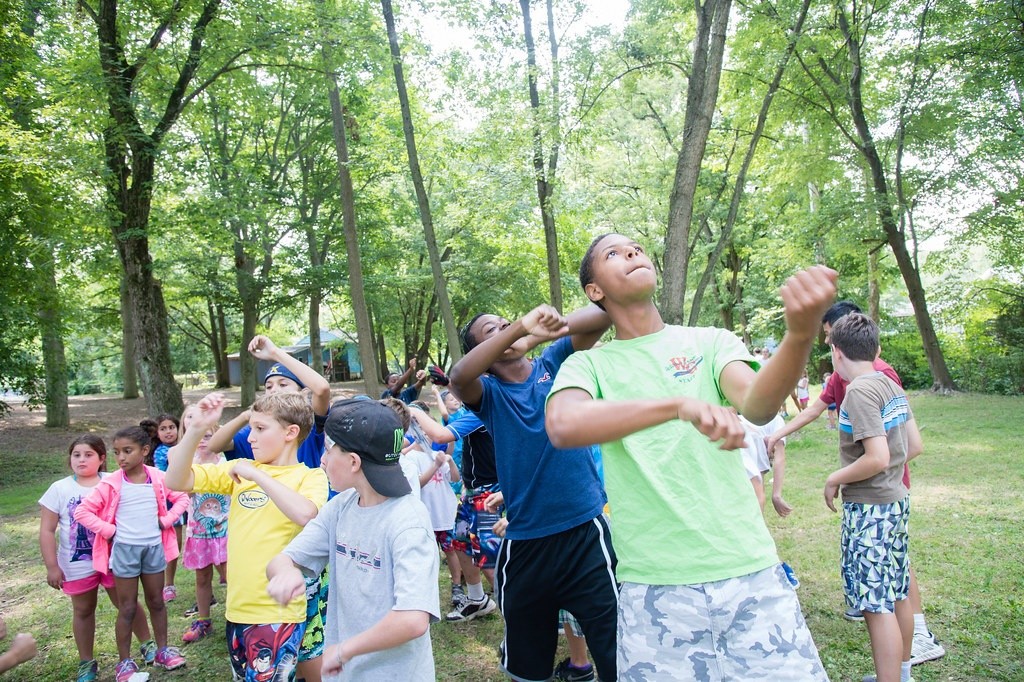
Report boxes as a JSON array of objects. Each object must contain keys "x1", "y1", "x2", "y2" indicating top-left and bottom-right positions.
[{"x1": 295, "y1": 328, "x2": 361, "y2": 380}]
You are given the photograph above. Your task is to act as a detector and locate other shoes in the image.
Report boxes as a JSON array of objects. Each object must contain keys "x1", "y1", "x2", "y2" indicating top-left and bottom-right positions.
[
  {"x1": 220, "y1": 581, "x2": 226, "y2": 586},
  {"x1": 553, "y1": 656, "x2": 594, "y2": 681},
  {"x1": 826, "y1": 424, "x2": 836, "y2": 431},
  {"x1": 862, "y1": 675, "x2": 914, "y2": 682},
  {"x1": 486, "y1": 589, "x2": 499, "y2": 599},
  {"x1": 783, "y1": 412, "x2": 788, "y2": 418},
  {"x1": 163, "y1": 587, "x2": 176, "y2": 601},
  {"x1": 449, "y1": 570, "x2": 467, "y2": 583}
]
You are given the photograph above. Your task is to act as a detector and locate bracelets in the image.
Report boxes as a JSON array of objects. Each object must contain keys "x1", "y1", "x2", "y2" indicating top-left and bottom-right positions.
[{"x1": 336, "y1": 641, "x2": 343, "y2": 666}]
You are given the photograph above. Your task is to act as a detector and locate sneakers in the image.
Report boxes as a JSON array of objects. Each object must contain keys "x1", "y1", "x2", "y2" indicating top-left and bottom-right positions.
[
  {"x1": 76, "y1": 659, "x2": 98, "y2": 681},
  {"x1": 445, "y1": 593, "x2": 497, "y2": 623},
  {"x1": 844, "y1": 607, "x2": 865, "y2": 621},
  {"x1": 909, "y1": 631, "x2": 945, "y2": 665},
  {"x1": 184, "y1": 595, "x2": 219, "y2": 618},
  {"x1": 153, "y1": 647, "x2": 186, "y2": 670},
  {"x1": 451, "y1": 583, "x2": 465, "y2": 606},
  {"x1": 115, "y1": 658, "x2": 139, "y2": 682},
  {"x1": 183, "y1": 617, "x2": 211, "y2": 641},
  {"x1": 776, "y1": 561, "x2": 800, "y2": 590},
  {"x1": 140, "y1": 639, "x2": 156, "y2": 663}
]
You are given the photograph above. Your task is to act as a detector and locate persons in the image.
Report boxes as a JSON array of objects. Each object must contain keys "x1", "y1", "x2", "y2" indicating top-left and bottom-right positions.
[
  {"x1": 74, "y1": 421, "x2": 190, "y2": 682},
  {"x1": 165, "y1": 335, "x2": 502, "y2": 682},
  {"x1": 448, "y1": 234, "x2": 840, "y2": 682},
  {"x1": 38, "y1": 434, "x2": 157, "y2": 682},
  {"x1": 154, "y1": 402, "x2": 227, "y2": 641},
  {"x1": 0, "y1": 616, "x2": 38, "y2": 676},
  {"x1": 762, "y1": 303, "x2": 945, "y2": 682}
]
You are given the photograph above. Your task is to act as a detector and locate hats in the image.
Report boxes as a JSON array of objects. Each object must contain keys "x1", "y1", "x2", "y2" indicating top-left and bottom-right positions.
[
  {"x1": 264, "y1": 360, "x2": 305, "y2": 390},
  {"x1": 325, "y1": 399, "x2": 412, "y2": 497}
]
